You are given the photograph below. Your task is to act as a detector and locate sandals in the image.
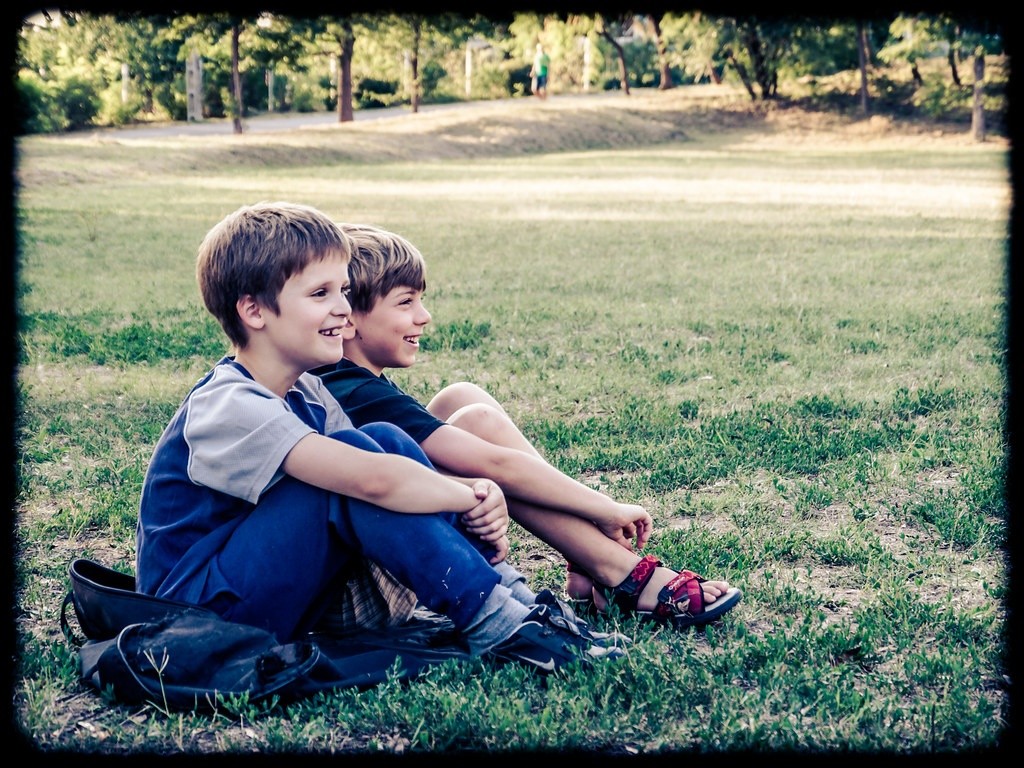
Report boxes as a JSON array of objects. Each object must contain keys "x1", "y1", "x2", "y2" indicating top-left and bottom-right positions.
[
  {"x1": 593, "y1": 554, "x2": 744, "y2": 628},
  {"x1": 563, "y1": 558, "x2": 634, "y2": 622}
]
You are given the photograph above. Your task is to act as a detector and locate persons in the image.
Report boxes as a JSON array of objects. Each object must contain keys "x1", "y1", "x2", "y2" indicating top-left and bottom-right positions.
[
  {"x1": 307, "y1": 226, "x2": 744, "y2": 625},
  {"x1": 530, "y1": 43, "x2": 551, "y2": 101},
  {"x1": 134, "y1": 202, "x2": 630, "y2": 676}
]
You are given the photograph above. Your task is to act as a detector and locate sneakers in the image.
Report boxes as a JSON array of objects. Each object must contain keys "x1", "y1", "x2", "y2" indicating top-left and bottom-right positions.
[
  {"x1": 480, "y1": 601, "x2": 622, "y2": 677},
  {"x1": 532, "y1": 588, "x2": 633, "y2": 647}
]
